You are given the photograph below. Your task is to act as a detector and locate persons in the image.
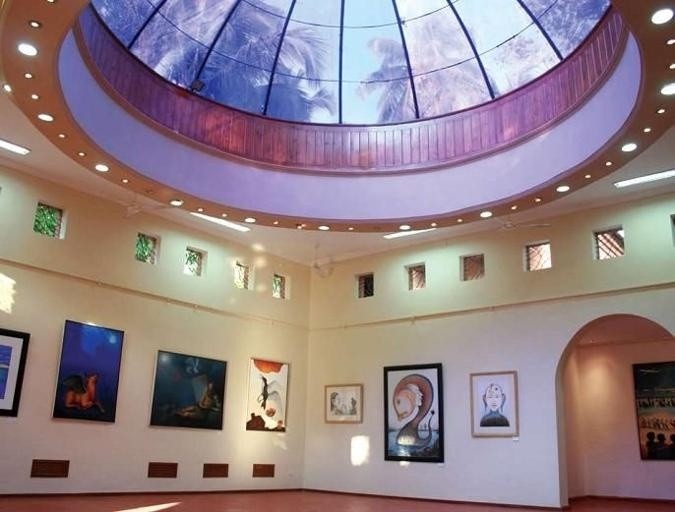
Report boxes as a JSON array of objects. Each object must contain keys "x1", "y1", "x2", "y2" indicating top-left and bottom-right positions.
[{"x1": 643, "y1": 430, "x2": 675, "y2": 460}]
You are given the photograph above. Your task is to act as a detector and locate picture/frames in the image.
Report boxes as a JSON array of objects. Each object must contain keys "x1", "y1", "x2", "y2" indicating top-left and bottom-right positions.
[
  {"x1": 324, "y1": 383, "x2": 363, "y2": 424},
  {"x1": 0, "y1": 326, "x2": 32, "y2": 418},
  {"x1": 468, "y1": 369, "x2": 520, "y2": 439},
  {"x1": 384, "y1": 363, "x2": 445, "y2": 462}
]
